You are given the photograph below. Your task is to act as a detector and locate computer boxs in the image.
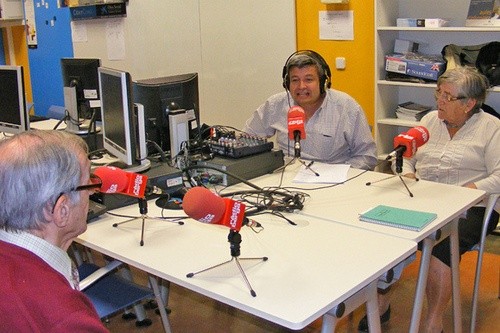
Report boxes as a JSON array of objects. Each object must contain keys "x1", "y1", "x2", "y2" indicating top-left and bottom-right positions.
[{"x1": 57, "y1": 129, "x2": 285, "y2": 212}]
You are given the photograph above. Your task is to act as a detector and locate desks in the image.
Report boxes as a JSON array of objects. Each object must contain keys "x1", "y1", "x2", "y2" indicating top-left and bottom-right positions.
[{"x1": 0, "y1": 119, "x2": 488, "y2": 333}]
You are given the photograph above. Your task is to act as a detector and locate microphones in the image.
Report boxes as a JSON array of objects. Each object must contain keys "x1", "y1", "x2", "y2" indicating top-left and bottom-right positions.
[
  {"x1": 385, "y1": 126, "x2": 430, "y2": 160},
  {"x1": 92, "y1": 167, "x2": 166, "y2": 199},
  {"x1": 182, "y1": 187, "x2": 262, "y2": 230},
  {"x1": 287, "y1": 106, "x2": 306, "y2": 156}
]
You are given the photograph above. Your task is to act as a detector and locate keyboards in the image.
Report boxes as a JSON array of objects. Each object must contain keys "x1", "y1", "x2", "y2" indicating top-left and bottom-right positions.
[{"x1": 87, "y1": 199, "x2": 107, "y2": 222}]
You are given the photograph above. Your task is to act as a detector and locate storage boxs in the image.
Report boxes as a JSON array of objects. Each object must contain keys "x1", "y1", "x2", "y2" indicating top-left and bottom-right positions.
[{"x1": 384, "y1": 52, "x2": 445, "y2": 80}]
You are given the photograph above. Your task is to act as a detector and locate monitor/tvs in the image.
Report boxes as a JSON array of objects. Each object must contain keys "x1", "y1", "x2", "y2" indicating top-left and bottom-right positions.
[
  {"x1": 98, "y1": 67, "x2": 151, "y2": 172},
  {"x1": 131, "y1": 73, "x2": 200, "y2": 154},
  {"x1": 61, "y1": 58, "x2": 102, "y2": 135},
  {"x1": 0, "y1": 65, "x2": 30, "y2": 135}
]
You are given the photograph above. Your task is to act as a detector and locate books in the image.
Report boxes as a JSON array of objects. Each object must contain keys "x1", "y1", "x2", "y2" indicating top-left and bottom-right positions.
[
  {"x1": 360, "y1": 205, "x2": 437, "y2": 232},
  {"x1": 397, "y1": 18, "x2": 448, "y2": 27},
  {"x1": 395, "y1": 101, "x2": 433, "y2": 121},
  {"x1": 384, "y1": 39, "x2": 448, "y2": 83},
  {"x1": 466, "y1": 0, "x2": 500, "y2": 27}
]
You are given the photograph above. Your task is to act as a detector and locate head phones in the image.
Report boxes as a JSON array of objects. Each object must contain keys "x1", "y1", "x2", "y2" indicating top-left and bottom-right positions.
[{"x1": 281, "y1": 50, "x2": 332, "y2": 94}]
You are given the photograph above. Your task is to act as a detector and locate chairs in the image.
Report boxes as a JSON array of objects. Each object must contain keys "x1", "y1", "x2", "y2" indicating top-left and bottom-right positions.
[
  {"x1": 469, "y1": 192, "x2": 500, "y2": 333},
  {"x1": 70, "y1": 245, "x2": 171, "y2": 332}
]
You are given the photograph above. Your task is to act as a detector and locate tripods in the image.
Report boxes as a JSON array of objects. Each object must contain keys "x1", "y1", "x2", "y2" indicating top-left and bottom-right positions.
[
  {"x1": 112, "y1": 197, "x2": 184, "y2": 246},
  {"x1": 269, "y1": 147, "x2": 320, "y2": 176},
  {"x1": 366, "y1": 154, "x2": 419, "y2": 198},
  {"x1": 186, "y1": 230, "x2": 268, "y2": 297}
]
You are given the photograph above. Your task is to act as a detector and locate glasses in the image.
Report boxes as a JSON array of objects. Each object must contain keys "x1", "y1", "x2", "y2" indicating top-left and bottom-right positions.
[
  {"x1": 433, "y1": 89, "x2": 466, "y2": 104},
  {"x1": 51, "y1": 173, "x2": 102, "y2": 215}
]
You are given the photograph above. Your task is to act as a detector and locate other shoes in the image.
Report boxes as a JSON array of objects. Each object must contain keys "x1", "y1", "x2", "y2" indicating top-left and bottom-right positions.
[{"x1": 358, "y1": 304, "x2": 390, "y2": 330}]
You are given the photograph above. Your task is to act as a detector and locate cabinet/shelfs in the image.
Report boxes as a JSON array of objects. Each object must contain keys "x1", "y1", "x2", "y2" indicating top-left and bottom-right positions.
[{"x1": 374, "y1": 1, "x2": 500, "y2": 231}]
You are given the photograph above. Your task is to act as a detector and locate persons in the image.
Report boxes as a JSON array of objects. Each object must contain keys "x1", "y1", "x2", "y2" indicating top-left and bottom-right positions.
[
  {"x1": 357, "y1": 65, "x2": 500, "y2": 333},
  {"x1": 0, "y1": 129, "x2": 111, "y2": 333},
  {"x1": 240, "y1": 52, "x2": 378, "y2": 171}
]
